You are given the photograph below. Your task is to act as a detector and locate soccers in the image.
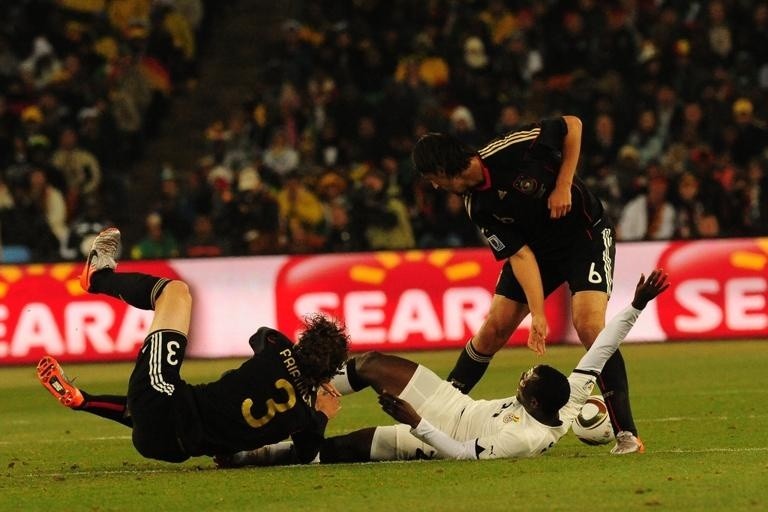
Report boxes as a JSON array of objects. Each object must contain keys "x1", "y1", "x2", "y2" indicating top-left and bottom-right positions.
[{"x1": 571, "y1": 395, "x2": 616, "y2": 445}]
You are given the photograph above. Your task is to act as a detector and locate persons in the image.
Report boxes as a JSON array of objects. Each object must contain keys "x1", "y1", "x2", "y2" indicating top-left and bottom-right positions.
[
  {"x1": 35, "y1": 226, "x2": 348, "y2": 465},
  {"x1": 1, "y1": 1, "x2": 768, "y2": 262},
  {"x1": 412, "y1": 113, "x2": 645, "y2": 455},
  {"x1": 228, "y1": 266, "x2": 672, "y2": 464}
]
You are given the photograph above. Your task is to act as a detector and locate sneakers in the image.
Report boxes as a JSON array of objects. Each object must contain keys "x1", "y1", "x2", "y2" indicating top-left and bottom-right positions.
[
  {"x1": 36, "y1": 357, "x2": 84, "y2": 408},
  {"x1": 213, "y1": 453, "x2": 236, "y2": 468},
  {"x1": 611, "y1": 431, "x2": 644, "y2": 455},
  {"x1": 79, "y1": 226, "x2": 121, "y2": 290}
]
